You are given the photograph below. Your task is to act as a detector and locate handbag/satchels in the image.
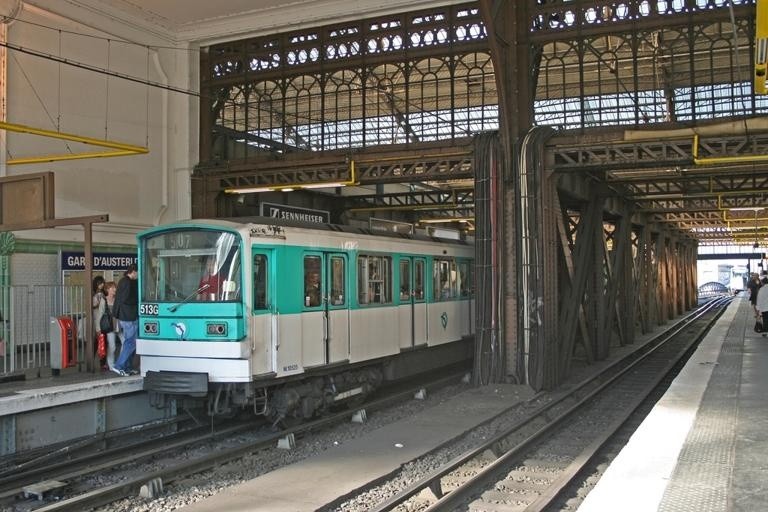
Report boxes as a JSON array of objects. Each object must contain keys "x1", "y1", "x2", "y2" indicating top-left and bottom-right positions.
[
  {"x1": 100, "y1": 295, "x2": 113, "y2": 335},
  {"x1": 753, "y1": 316, "x2": 764, "y2": 334},
  {"x1": 96, "y1": 333, "x2": 106, "y2": 359}
]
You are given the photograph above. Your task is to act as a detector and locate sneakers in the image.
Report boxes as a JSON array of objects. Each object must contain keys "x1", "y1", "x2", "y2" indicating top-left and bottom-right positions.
[
  {"x1": 99, "y1": 361, "x2": 139, "y2": 377},
  {"x1": 762, "y1": 333, "x2": 767, "y2": 338}
]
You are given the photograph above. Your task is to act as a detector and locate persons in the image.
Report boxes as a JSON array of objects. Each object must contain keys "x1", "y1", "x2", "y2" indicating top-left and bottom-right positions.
[
  {"x1": 91, "y1": 263, "x2": 139, "y2": 378},
  {"x1": 196, "y1": 257, "x2": 226, "y2": 301},
  {"x1": 747, "y1": 270, "x2": 768, "y2": 338},
  {"x1": 303, "y1": 262, "x2": 458, "y2": 306}
]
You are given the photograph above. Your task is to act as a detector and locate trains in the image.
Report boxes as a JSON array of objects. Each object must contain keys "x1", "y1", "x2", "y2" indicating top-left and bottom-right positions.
[{"x1": 135, "y1": 217, "x2": 476, "y2": 431}]
